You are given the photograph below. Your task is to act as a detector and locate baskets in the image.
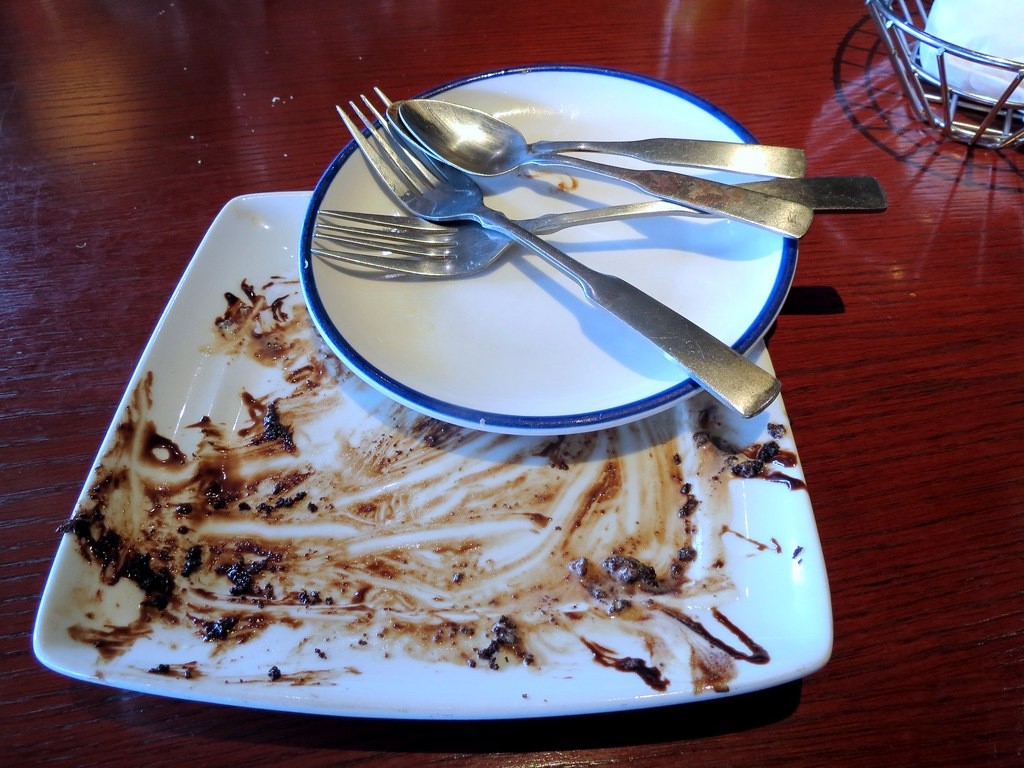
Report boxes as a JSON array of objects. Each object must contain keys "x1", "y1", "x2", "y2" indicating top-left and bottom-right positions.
[{"x1": 866, "y1": 0, "x2": 1023, "y2": 148}]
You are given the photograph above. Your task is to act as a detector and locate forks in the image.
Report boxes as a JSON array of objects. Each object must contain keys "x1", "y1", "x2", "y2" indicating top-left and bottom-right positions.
[
  {"x1": 334, "y1": 85, "x2": 781, "y2": 419},
  {"x1": 309, "y1": 177, "x2": 888, "y2": 277}
]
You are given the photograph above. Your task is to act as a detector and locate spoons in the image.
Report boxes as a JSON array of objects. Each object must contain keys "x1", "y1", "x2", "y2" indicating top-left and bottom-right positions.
[{"x1": 386, "y1": 97, "x2": 814, "y2": 238}]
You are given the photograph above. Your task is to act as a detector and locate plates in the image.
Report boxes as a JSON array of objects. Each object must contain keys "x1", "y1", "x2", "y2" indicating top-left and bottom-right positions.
[
  {"x1": 33, "y1": 188, "x2": 835, "y2": 721},
  {"x1": 298, "y1": 64, "x2": 799, "y2": 435}
]
